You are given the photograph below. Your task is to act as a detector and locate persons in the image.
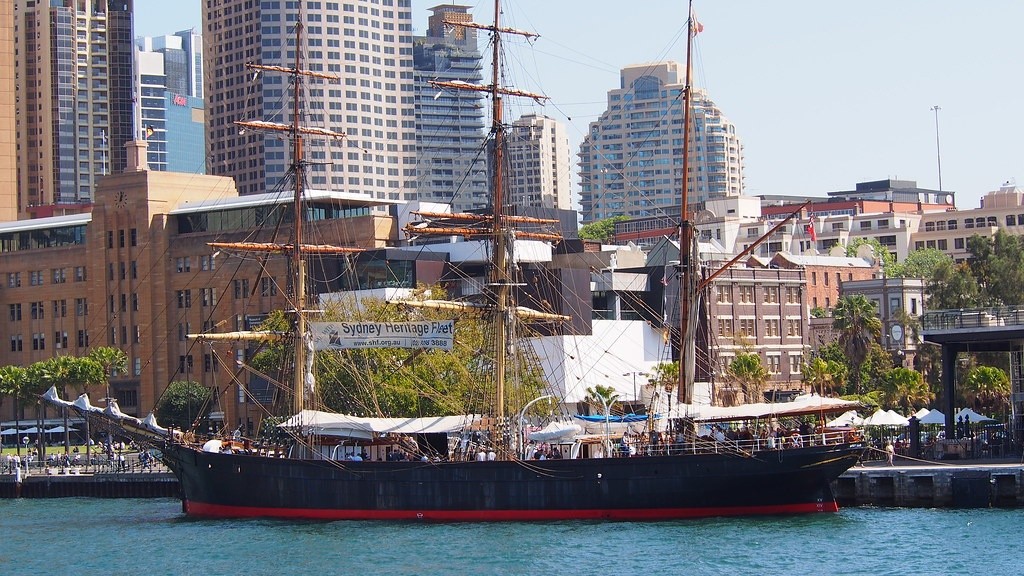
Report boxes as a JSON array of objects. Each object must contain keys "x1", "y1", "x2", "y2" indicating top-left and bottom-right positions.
[
  {"x1": 854, "y1": 457, "x2": 864, "y2": 467},
  {"x1": 347, "y1": 442, "x2": 563, "y2": 461},
  {"x1": 5, "y1": 425, "x2": 193, "y2": 471},
  {"x1": 619, "y1": 421, "x2": 815, "y2": 458},
  {"x1": 886, "y1": 442, "x2": 894, "y2": 466}
]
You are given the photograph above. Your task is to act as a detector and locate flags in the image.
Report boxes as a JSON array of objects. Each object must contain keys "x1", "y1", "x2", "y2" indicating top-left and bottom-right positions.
[{"x1": 692, "y1": 8, "x2": 703, "y2": 37}]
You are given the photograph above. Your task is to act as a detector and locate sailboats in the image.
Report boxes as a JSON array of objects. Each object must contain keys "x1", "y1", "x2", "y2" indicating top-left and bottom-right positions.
[{"x1": 30, "y1": 0, "x2": 873, "y2": 517}]
